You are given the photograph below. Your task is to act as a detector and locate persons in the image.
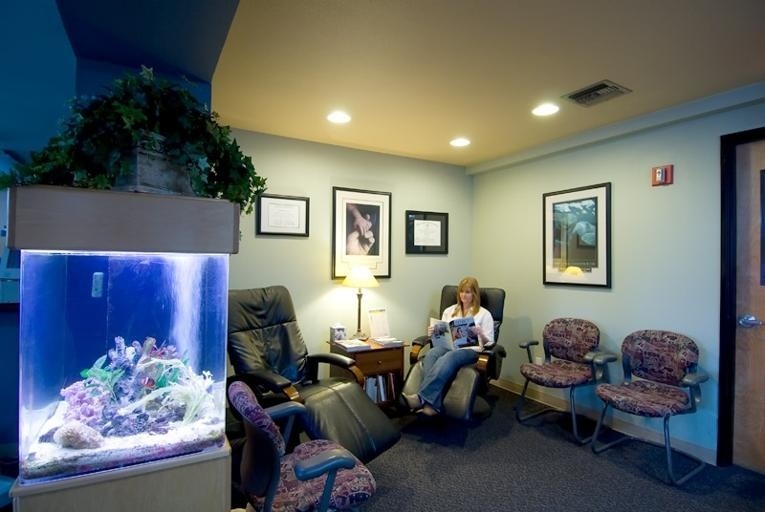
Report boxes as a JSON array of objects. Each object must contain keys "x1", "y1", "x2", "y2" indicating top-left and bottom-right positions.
[
  {"x1": 347, "y1": 204, "x2": 372, "y2": 235},
  {"x1": 346, "y1": 230, "x2": 375, "y2": 255},
  {"x1": 401, "y1": 276, "x2": 495, "y2": 418}
]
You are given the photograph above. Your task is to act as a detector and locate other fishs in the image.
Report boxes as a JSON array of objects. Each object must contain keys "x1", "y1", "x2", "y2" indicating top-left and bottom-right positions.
[
  {"x1": 140, "y1": 336, "x2": 158, "y2": 358},
  {"x1": 140, "y1": 377, "x2": 157, "y2": 389}
]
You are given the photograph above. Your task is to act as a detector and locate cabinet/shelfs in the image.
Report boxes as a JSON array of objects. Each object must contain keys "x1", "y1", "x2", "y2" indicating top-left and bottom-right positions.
[{"x1": 326, "y1": 338, "x2": 410, "y2": 412}]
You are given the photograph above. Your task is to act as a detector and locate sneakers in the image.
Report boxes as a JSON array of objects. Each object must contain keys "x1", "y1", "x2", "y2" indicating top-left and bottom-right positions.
[
  {"x1": 416, "y1": 405, "x2": 437, "y2": 416},
  {"x1": 401, "y1": 391, "x2": 421, "y2": 413}
]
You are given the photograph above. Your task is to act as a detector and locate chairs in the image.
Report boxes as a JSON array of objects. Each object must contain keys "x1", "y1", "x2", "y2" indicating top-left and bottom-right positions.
[
  {"x1": 397, "y1": 282, "x2": 510, "y2": 421},
  {"x1": 227, "y1": 380, "x2": 378, "y2": 510},
  {"x1": 592, "y1": 327, "x2": 705, "y2": 485},
  {"x1": 516, "y1": 317, "x2": 610, "y2": 445},
  {"x1": 227, "y1": 287, "x2": 401, "y2": 469}
]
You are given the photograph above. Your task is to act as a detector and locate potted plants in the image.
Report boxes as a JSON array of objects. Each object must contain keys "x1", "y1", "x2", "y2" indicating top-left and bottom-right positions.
[{"x1": 0, "y1": 66, "x2": 267, "y2": 214}]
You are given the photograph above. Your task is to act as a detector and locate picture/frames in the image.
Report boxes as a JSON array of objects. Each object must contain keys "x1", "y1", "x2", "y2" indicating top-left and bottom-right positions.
[
  {"x1": 255, "y1": 193, "x2": 310, "y2": 236},
  {"x1": 331, "y1": 185, "x2": 393, "y2": 279},
  {"x1": 543, "y1": 182, "x2": 613, "y2": 291},
  {"x1": 405, "y1": 210, "x2": 449, "y2": 255}
]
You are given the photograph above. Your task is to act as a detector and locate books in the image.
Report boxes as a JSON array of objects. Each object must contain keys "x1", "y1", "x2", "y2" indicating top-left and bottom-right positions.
[
  {"x1": 334, "y1": 337, "x2": 404, "y2": 354},
  {"x1": 430, "y1": 315, "x2": 480, "y2": 351}
]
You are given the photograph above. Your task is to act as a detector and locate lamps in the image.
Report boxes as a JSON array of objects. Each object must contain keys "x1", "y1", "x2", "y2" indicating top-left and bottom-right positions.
[{"x1": 340, "y1": 265, "x2": 379, "y2": 340}]
